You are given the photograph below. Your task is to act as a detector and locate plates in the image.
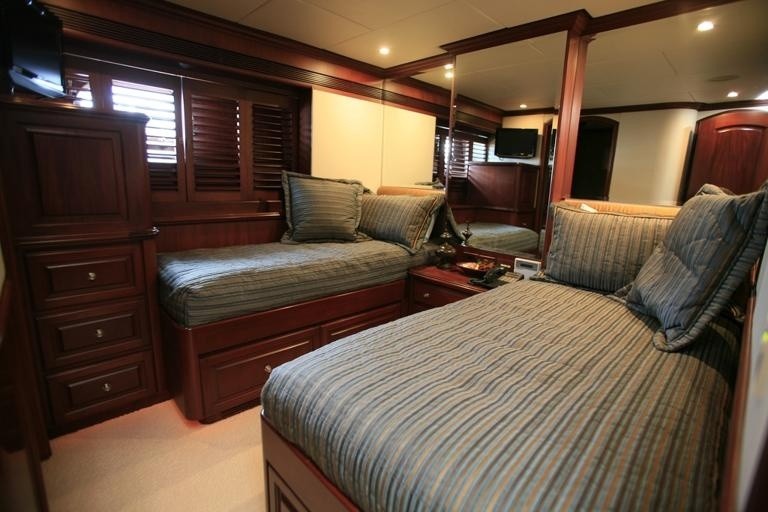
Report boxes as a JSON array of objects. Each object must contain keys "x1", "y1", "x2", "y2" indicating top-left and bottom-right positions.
[{"x1": 457, "y1": 260, "x2": 504, "y2": 277}]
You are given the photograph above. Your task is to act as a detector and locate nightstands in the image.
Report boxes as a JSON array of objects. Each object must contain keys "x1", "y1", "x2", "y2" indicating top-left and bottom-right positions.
[{"x1": 407, "y1": 256, "x2": 494, "y2": 314}]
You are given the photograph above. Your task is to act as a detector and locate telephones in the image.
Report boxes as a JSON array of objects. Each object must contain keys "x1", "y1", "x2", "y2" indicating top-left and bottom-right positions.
[{"x1": 480, "y1": 266, "x2": 524, "y2": 288}]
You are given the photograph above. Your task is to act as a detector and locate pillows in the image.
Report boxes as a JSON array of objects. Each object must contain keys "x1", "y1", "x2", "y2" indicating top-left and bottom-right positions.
[{"x1": 280, "y1": 170, "x2": 446, "y2": 255}]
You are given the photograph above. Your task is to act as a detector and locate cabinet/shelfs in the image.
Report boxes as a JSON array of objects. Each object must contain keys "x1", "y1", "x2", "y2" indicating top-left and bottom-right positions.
[
  {"x1": 1, "y1": 98, "x2": 154, "y2": 240},
  {"x1": 198, "y1": 323, "x2": 323, "y2": 418},
  {"x1": 0, "y1": 238, "x2": 168, "y2": 461},
  {"x1": 466, "y1": 209, "x2": 535, "y2": 229},
  {"x1": 466, "y1": 161, "x2": 541, "y2": 213},
  {"x1": 320, "y1": 302, "x2": 402, "y2": 346}
]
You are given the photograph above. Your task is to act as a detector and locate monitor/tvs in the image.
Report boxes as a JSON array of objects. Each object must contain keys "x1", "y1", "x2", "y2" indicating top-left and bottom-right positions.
[
  {"x1": 495, "y1": 128, "x2": 538, "y2": 158},
  {"x1": 0, "y1": 0, "x2": 69, "y2": 101}
]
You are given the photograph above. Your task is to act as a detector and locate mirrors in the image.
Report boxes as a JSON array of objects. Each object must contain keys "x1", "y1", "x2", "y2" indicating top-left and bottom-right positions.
[
  {"x1": 380, "y1": 53, "x2": 453, "y2": 190},
  {"x1": 561, "y1": 0, "x2": 768, "y2": 205},
  {"x1": 438, "y1": 8, "x2": 593, "y2": 268}
]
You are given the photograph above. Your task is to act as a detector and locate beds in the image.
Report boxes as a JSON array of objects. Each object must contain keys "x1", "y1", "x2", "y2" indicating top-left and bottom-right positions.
[
  {"x1": 261, "y1": 198, "x2": 768, "y2": 512},
  {"x1": 156, "y1": 184, "x2": 446, "y2": 424},
  {"x1": 441, "y1": 221, "x2": 539, "y2": 251}
]
